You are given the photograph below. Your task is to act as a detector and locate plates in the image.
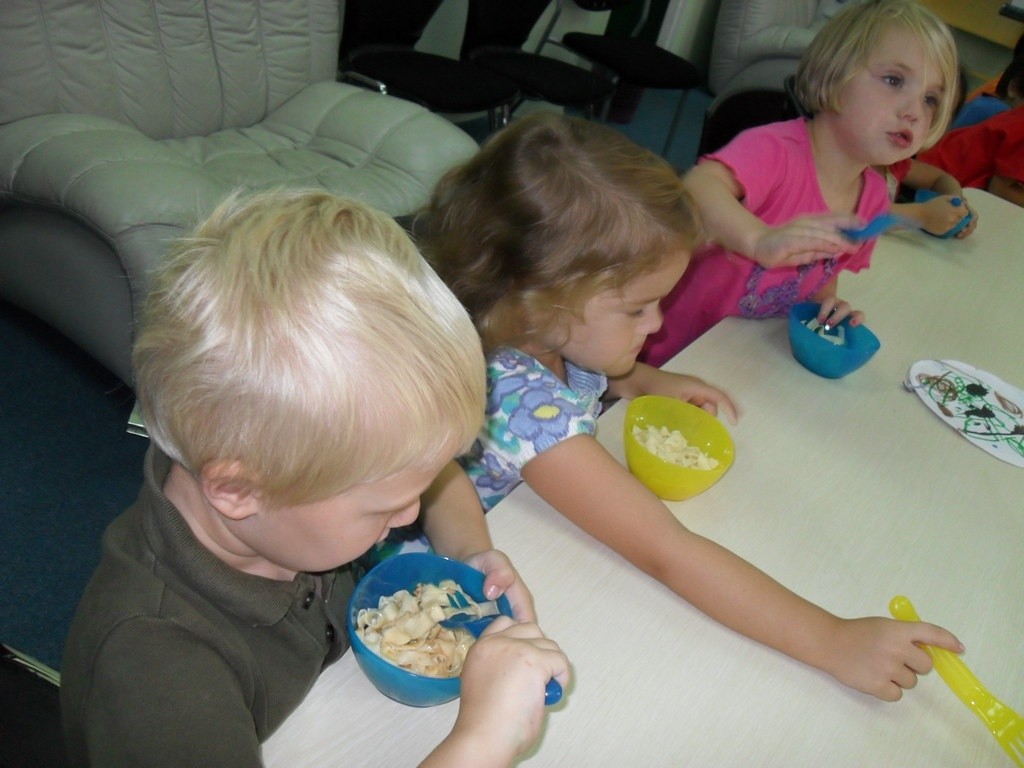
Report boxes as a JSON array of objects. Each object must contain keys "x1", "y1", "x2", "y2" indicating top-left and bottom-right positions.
[{"x1": 908, "y1": 359, "x2": 1024, "y2": 468}]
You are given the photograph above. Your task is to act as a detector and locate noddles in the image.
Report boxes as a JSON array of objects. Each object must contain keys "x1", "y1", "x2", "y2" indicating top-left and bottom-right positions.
[
  {"x1": 632, "y1": 425, "x2": 718, "y2": 471},
  {"x1": 355, "y1": 578, "x2": 500, "y2": 677}
]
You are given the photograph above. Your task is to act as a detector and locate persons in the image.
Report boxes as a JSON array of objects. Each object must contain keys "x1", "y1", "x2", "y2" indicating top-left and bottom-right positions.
[
  {"x1": 638, "y1": 0, "x2": 958, "y2": 369},
  {"x1": 867, "y1": 32, "x2": 1024, "y2": 238},
  {"x1": 59, "y1": 187, "x2": 574, "y2": 768},
  {"x1": 408, "y1": 114, "x2": 967, "y2": 702}
]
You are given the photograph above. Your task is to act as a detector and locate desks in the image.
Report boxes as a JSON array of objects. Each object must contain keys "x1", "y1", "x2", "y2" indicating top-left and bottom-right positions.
[{"x1": 259, "y1": 187, "x2": 1024, "y2": 768}]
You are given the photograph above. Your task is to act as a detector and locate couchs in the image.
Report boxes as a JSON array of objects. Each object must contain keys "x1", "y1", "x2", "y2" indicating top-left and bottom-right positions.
[
  {"x1": 0, "y1": 0, "x2": 480, "y2": 390},
  {"x1": 695, "y1": 0, "x2": 851, "y2": 168}
]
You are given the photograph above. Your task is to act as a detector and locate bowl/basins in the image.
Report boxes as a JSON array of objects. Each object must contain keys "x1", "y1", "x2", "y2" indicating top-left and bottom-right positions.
[
  {"x1": 913, "y1": 188, "x2": 972, "y2": 239},
  {"x1": 786, "y1": 302, "x2": 880, "y2": 379},
  {"x1": 347, "y1": 553, "x2": 513, "y2": 708},
  {"x1": 625, "y1": 395, "x2": 735, "y2": 502}
]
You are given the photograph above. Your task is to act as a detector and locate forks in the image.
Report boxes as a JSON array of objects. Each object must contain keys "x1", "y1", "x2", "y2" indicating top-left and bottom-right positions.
[
  {"x1": 888, "y1": 594, "x2": 1024, "y2": 768},
  {"x1": 837, "y1": 211, "x2": 921, "y2": 242},
  {"x1": 436, "y1": 586, "x2": 563, "y2": 707}
]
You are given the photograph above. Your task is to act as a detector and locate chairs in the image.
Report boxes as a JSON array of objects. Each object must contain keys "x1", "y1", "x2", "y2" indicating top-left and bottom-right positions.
[{"x1": 337, "y1": 0, "x2": 709, "y2": 140}]
